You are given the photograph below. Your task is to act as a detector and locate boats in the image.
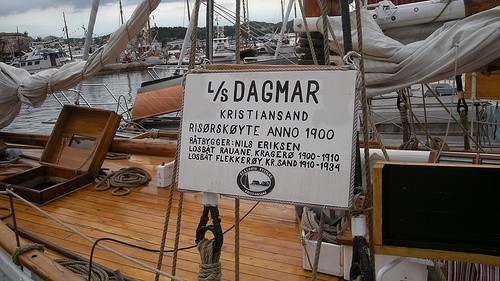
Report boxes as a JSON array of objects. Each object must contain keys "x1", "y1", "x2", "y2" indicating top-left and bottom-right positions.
[{"x1": 0, "y1": 0, "x2": 499, "y2": 281}]
